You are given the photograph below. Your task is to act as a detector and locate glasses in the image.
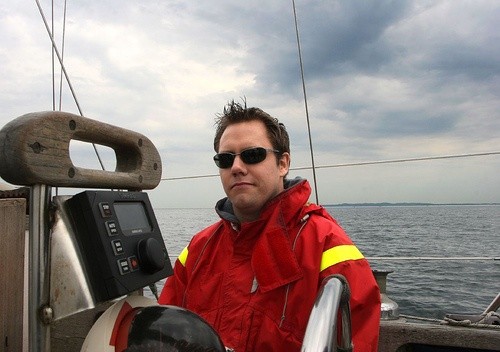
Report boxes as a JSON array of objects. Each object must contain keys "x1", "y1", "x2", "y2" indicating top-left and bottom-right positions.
[{"x1": 212, "y1": 146, "x2": 283, "y2": 168}]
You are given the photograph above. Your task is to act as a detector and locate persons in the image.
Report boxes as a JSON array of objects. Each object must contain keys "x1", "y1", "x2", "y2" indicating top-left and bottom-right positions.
[{"x1": 157, "y1": 95, "x2": 381, "y2": 352}]
